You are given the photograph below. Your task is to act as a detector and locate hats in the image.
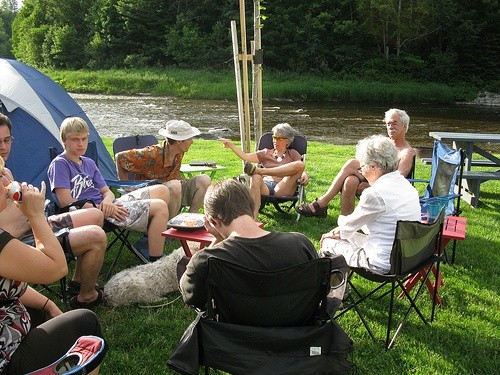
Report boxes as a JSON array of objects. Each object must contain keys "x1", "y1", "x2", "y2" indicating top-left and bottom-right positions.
[{"x1": 158, "y1": 119, "x2": 202, "y2": 141}]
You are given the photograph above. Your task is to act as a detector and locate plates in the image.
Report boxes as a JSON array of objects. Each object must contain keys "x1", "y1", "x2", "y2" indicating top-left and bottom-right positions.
[{"x1": 167, "y1": 212, "x2": 208, "y2": 230}]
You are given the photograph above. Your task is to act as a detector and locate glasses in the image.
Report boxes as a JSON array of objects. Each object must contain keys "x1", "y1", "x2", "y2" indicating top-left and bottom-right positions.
[
  {"x1": 385, "y1": 122, "x2": 402, "y2": 129},
  {"x1": 272, "y1": 135, "x2": 289, "y2": 143},
  {"x1": 0, "y1": 135, "x2": 15, "y2": 145},
  {"x1": 356, "y1": 165, "x2": 365, "y2": 173}
]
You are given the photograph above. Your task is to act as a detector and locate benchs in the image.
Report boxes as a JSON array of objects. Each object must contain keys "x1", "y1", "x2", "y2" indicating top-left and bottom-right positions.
[
  {"x1": 441, "y1": 170, "x2": 500, "y2": 208},
  {"x1": 422, "y1": 158, "x2": 500, "y2": 166}
]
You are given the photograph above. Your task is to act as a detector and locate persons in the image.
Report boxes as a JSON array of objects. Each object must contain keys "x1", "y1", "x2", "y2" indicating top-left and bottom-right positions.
[
  {"x1": 0, "y1": 157, "x2": 109, "y2": 375},
  {"x1": 165, "y1": 179, "x2": 358, "y2": 375},
  {"x1": 224, "y1": 123, "x2": 308, "y2": 220},
  {"x1": 114, "y1": 120, "x2": 211, "y2": 218},
  {"x1": 295, "y1": 108, "x2": 415, "y2": 218},
  {"x1": 320, "y1": 135, "x2": 421, "y2": 308},
  {"x1": 47, "y1": 117, "x2": 169, "y2": 260},
  {"x1": 0, "y1": 112, "x2": 106, "y2": 306}
]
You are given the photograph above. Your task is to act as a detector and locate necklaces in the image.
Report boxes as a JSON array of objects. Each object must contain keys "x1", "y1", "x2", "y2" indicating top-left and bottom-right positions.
[{"x1": 275, "y1": 151, "x2": 286, "y2": 162}]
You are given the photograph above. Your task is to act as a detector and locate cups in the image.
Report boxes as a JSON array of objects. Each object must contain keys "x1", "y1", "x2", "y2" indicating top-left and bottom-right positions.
[{"x1": 421, "y1": 203, "x2": 447, "y2": 222}]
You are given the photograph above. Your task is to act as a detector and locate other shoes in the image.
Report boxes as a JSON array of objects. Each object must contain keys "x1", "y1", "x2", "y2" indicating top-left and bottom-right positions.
[
  {"x1": 132, "y1": 236, "x2": 151, "y2": 264},
  {"x1": 69, "y1": 288, "x2": 114, "y2": 309},
  {"x1": 244, "y1": 161, "x2": 256, "y2": 177},
  {"x1": 66, "y1": 280, "x2": 103, "y2": 295}
]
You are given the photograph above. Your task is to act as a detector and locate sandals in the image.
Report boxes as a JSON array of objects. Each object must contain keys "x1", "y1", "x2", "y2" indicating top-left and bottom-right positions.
[{"x1": 295, "y1": 197, "x2": 328, "y2": 218}]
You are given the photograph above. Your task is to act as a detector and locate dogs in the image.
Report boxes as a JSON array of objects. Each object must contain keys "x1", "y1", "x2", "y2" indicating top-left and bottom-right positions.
[{"x1": 102, "y1": 240, "x2": 207, "y2": 307}]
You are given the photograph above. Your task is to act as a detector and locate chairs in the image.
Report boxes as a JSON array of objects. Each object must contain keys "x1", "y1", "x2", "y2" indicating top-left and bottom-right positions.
[{"x1": 15, "y1": 132, "x2": 446, "y2": 375}]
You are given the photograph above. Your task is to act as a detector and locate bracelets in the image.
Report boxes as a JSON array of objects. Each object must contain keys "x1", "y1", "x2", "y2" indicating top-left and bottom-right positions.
[{"x1": 43, "y1": 298, "x2": 49, "y2": 309}]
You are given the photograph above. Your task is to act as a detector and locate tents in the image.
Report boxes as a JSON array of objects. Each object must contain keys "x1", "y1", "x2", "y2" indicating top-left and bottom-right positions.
[{"x1": 0, "y1": 58, "x2": 116, "y2": 214}]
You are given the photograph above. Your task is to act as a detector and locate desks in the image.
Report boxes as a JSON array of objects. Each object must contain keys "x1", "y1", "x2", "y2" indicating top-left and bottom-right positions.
[
  {"x1": 429, "y1": 132, "x2": 500, "y2": 208},
  {"x1": 396, "y1": 213, "x2": 468, "y2": 308},
  {"x1": 179, "y1": 163, "x2": 227, "y2": 179},
  {"x1": 160, "y1": 222, "x2": 264, "y2": 255}
]
